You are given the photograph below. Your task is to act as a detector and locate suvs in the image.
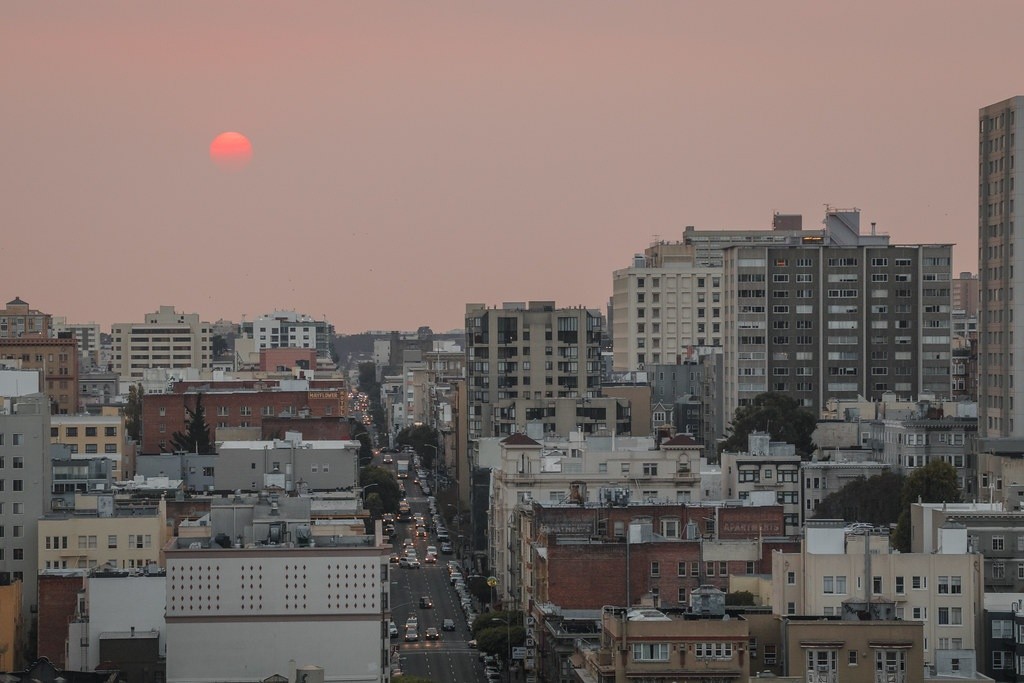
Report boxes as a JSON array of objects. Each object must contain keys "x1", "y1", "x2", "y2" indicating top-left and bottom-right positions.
[
  {"x1": 399, "y1": 556, "x2": 421, "y2": 569},
  {"x1": 389, "y1": 622, "x2": 398, "y2": 639},
  {"x1": 420, "y1": 596, "x2": 433, "y2": 610},
  {"x1": 405, "y1": 617, "x2": 419, "y2": 630},
  {"x1": 405, "y1": 628, "x2": 418, "y2": 642}
]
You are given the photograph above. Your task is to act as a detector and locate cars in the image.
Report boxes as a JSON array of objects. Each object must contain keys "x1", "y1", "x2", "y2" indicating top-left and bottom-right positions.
[
  {"x1": 348, "y1": 391, "x2": 453, "y2": 556},
  {"x1": 391, "y1": 644, "x2": 403, "y2": 679},
  {"x1": 442, "y1": 618, "x2": 458, "y2": 631},
  {"x1": 425, "y1": 554, "x2": 437, "y2": 563},
  {"x1": 426, "y1": 627, "x2": 440, "y2": 640},
  {"x1": 389, "y1": 552, "x2": 400, "y2": 562},
  {"x1": 447, "y1": 560, "x2": 502, "y2": 683}
]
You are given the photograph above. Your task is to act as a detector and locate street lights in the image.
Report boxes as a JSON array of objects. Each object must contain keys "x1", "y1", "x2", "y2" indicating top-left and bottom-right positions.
[
  {"x1": 491, "y1": 618, "x2": 511, "y2": 662},
  {"x1": 425, "y1": 443, "x2": 439, "y2": 494}
]
